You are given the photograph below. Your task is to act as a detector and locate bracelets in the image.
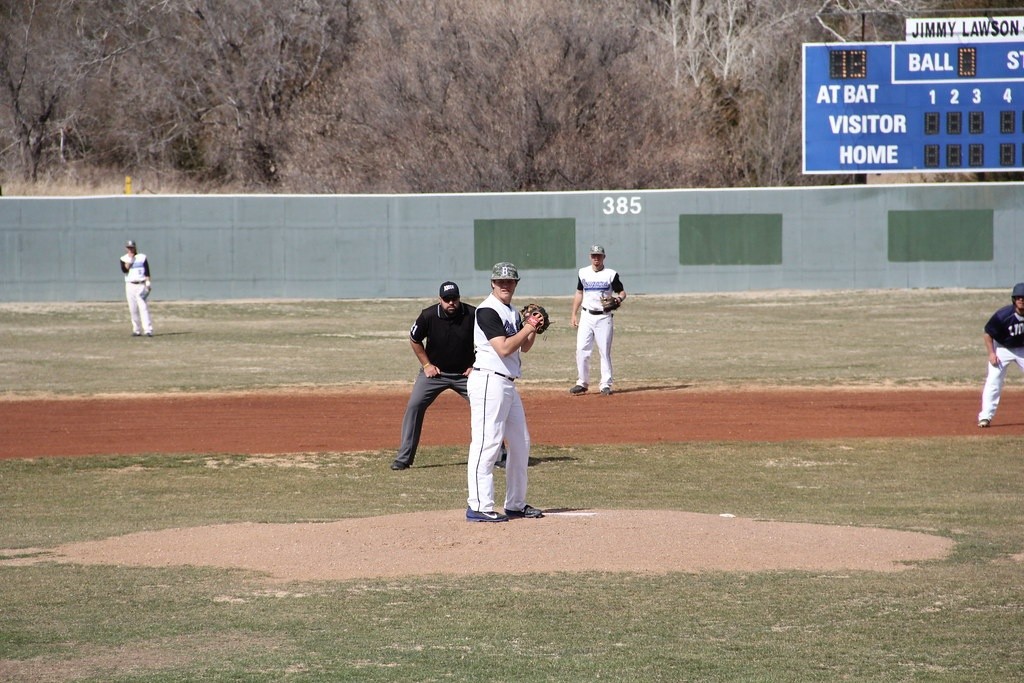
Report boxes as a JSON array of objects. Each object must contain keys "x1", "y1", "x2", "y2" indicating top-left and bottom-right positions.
[{"x1": 423, "y1": 361, "x2": 430, "y2": 369}]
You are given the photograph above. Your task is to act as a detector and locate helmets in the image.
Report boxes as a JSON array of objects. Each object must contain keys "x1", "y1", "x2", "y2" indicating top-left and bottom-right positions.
[{"x1": 1011, "y1": 283, "x2": 1024, "y2": 297}]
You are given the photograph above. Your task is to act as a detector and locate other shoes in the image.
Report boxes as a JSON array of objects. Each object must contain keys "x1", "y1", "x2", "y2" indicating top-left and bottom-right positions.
[
  {"x1": 391, "y1": 460, "x2": 406, "y2": 470},
  {"x1": 145, "y1": 331, "x2": 152, "y2": 336},
  {"x1": 130, "y1": 332, "x2": 141, "y2": 336},
  {"x1": 601, "y1": 387, "x2": 610, "y2": 393},
  {"x1": 570, "y1": 385, "x2": 588, "y2": 395}
]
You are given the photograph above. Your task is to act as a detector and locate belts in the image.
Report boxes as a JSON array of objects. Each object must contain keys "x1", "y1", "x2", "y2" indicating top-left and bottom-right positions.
[
  {"x1": 131, "y1": 281, "x2": 145, "y2": 284},
  {"x1": 583, "y1": 307, "x2": 603, "y2": 315},
  {"x1": 474, "y1": 367, "x2": 515, "y2": 382}
]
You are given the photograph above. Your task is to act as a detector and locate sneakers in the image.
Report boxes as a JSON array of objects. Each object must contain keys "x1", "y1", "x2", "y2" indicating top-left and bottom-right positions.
[
  {"x1": 466, "y1": 505, "x2": 509, "y2": 522},
  {"x1": 504, "y1": 505, "x2": 542, "y2": 519},
  {"x1": 978, "y1": 419, "x2": 990, "y2": 428}
]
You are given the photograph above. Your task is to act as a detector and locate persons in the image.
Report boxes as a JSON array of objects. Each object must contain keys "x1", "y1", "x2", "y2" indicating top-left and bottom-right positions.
[
  {"x1": 467, "y1": 262, "x2": 551, "y2": 523},
  {"x1": 120, "y1": 239, "x2": 153, "y2": 337},
  {"x1": 391, "y1": 280, "x2": 506, "y2": 471},
  {"x1": 570, "y1": 245, "x2": 626, "y2": 395},
  {"x1": 977, "y1": 282, "x2": 1024, "y2": 428}
]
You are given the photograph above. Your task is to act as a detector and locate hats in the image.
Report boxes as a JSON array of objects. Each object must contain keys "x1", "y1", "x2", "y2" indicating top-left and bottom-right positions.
[
  {"x1": 125, "y1": 241, "x2": 135, "y2": 247},
  {"x1": 440, "y1": 281, "x2": 459, "y2": 297},
  {"x1": 589, "y1": 244, "x2": 604, "y2": 254},
  {"x1": 490, "y1": 262, "x2": 520, "y2": 280}
]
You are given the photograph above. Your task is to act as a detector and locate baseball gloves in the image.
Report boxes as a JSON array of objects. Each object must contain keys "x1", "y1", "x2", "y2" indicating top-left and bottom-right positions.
[
  {"x1": 519, "y1": 303, "x2": 549, "y2": 334},
  {"x1": 601, "y1": 297, "x2": 619, "y2": 313},
  {"x1": 141, "y1": 286, "x2": 151, "y2": 300}
]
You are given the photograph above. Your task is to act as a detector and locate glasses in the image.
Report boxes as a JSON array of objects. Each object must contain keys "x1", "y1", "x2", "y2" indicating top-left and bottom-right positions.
[{"x1": 441, "y1": 295, "x2": 459, "y2": 302}]
